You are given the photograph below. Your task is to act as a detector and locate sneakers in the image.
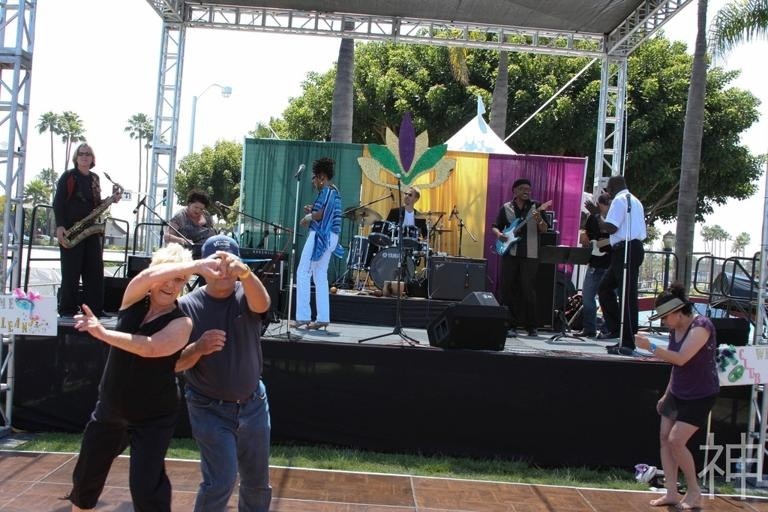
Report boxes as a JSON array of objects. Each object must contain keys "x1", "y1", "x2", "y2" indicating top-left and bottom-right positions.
[
  {"x1": 528, "y1": 329, "x2": 537, "y2": 336},
  {"x1": 507, "y1": 328, "x2": 516, "y2": 337},
  {"x1": 573, "y1": 328, "x2": 596, "y2": 337},
  {"x1": 634, "y1": 463, "x2": 683, "y2": 489}
]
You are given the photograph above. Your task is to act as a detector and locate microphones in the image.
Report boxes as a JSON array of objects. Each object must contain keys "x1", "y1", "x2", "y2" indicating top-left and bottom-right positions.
[
  {"x1": 133, "y1": 196, "x2": 146, "y2": 214},
  {"x1": 626, "y1": 194, "x2": 632, "y2": 212},
  {"x1": 216, "y1": 201, "x2": 230, "y2": 211},
  {"x1": 294, "y1": 164, "x2": 306, "y2": 178},
  {"x1": 396, "y1": 174, "x2": 402, "y2": 183}
]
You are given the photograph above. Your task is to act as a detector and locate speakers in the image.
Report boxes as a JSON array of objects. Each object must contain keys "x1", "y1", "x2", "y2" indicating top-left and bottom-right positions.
[
  {"x1": 462, "y1": 291, "x2": 499, "y2": 305},
  {"x1": 711, "y1": 317, "x2": 750, "y2": 346},
  {"x1": 431, "y1": 258, "x2": 487, "y2": 299},
  {"x1": 427, "y1": 304, "x2": 509, "y2": 351}
]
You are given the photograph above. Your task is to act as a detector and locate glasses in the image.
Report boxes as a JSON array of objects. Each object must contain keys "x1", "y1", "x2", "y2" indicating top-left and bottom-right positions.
[{"x1": 78, "y1": 152, "x2": 90, "y2": 157}]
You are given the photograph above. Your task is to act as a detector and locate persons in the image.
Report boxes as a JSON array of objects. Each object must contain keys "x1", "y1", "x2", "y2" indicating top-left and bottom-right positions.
[
  {"x1": 289, "y1": 156, "x2": 342, "y2": 332},
  {"x1": 162, "y1": 189, "x2": 215, "y2": 247},
  {"x1": 65, "y1": 243, "x2": 225, "y2": 511},
  {"x1": 572, "y1": 190, "x2": 620, "y2": 339},
  {"x1": 383, "y1": 185, "x2": 432, "y2": 244},
  {"x1": 52, "y1": 143, "x2": 122, "y2": 319},
  {"x1": 581, "y1": 174, "x2": 646, "y2": 353},
  {"x1": 632, "y1": 283, "x2": 720, "y2": 510},
  {"x1": 177, "y1": 232, "x2": 275, "y2": 511},
  {"x1": 491, "y1": 177, "x2": 552, "y2": 338}
]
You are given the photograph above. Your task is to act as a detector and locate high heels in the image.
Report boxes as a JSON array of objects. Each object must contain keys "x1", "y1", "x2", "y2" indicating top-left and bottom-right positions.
[
  {"x1": 289, "y1": 320, "x2": 310, "y2": 329},
  {"x1": 307, "y1": 322, "x2": 329, "y2": 331}
]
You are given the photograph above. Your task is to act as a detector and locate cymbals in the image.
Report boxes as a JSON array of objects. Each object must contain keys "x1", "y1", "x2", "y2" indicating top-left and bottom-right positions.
[
  {"x1": 345, "y1": 208, "x2": 382, "y2": 225},
  {"x1": 414, "y1": 212, "x2": 446, "y2": 218}
]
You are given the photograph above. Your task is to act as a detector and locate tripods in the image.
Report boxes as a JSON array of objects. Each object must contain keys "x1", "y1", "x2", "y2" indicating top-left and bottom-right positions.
[
  {"x1": 551, "y1": 265, "x2": 585, "y2": 342},
  {"x1": 358, "y1": 199, "x2": 419, "y2": 345}
]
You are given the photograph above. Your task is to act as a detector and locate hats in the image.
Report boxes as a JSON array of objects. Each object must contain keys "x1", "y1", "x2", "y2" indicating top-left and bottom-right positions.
[
  {"x1": 512, "y1": 179, "x2": 531, "y2": 192},
  {"x1": 201, "y1": 235, "x2": 240, "y2": 257},
  {"x1": 649, "y1": 297, "x2": 684, "y2": 321}
]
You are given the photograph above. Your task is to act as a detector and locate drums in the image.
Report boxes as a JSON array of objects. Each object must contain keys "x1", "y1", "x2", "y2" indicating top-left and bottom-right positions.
[
  {"x1": 369, "y1": 221, "x2": 397, "y2": 246},
  {"x1": 399, "y1": 225, "x2": 422, "y2": 250},
  {"x1": 370, "y1": 248, "x2": 415, "y2": 290},
  {"x1": 346, "y1": 236, "x2": 379, "y2": 271}
]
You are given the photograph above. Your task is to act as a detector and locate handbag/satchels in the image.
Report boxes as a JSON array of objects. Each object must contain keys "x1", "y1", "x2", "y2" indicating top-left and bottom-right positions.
[{"x1": 405, "y1": 268, "x2": 428, "y2": 297}]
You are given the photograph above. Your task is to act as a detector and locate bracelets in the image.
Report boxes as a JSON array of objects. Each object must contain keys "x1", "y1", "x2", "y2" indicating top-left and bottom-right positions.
[
  {"x1": 594, "y1": 212, "x2": 602, "y2": 219},
  {"x1": 648, "y1": 341, "x2": 656, "y2": 353},
  {"x1": 236, "y1": 263, "x2": 252, "y2": 280},
  {"x1": 536, "y1": 217, "x2": 544, "y2": 225}
]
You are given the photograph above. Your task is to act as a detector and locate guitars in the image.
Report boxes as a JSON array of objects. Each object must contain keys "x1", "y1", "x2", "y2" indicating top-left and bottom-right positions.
[
  {"x1": 584, "y1": 238, "x2": 611, "y2": 257},
  {"x1": 495, "y1": 200, "x2": 551, "y2": 256}
]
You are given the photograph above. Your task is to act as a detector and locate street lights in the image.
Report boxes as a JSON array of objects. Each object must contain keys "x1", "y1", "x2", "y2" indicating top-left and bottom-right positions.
[
  {"x1": 189, "y1": 82, "x2": 235, "y2": 166},
  {"x1": 660, "y1": 233, "x2": 678, "y2": 293}
]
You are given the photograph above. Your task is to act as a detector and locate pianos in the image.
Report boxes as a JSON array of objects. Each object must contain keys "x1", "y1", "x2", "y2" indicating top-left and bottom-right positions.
[{"x1": 184, "y1": 245, "x2": 288, "y2": 262}]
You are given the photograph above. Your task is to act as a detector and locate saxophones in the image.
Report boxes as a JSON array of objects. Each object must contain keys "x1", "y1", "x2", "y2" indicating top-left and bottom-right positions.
[{"x1": 62, "y1": 173, "x2": 124, "y2": 249}]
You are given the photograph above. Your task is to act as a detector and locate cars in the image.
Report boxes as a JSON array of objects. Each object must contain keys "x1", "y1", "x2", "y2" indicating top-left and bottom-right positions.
[{"x1": 7, "y1": 231, "x2": 55, "y2": 245}]
